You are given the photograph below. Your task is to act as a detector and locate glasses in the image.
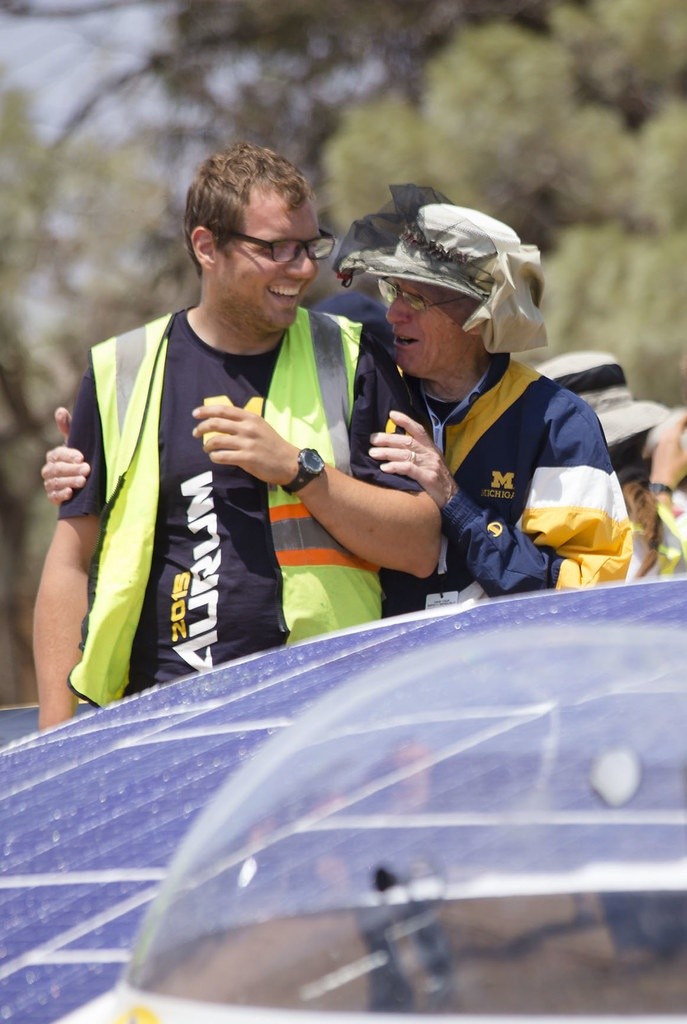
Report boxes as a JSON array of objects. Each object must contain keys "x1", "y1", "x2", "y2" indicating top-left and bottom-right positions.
[
  {"x1": 377, "y1": 277, "x2": 469, "y2": 311},
  {"x1": 206, "y1": 223, "x2": 336, "y2": 262}
]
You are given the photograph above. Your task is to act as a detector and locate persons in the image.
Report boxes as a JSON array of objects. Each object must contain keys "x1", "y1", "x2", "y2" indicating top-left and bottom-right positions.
[
  {"x1": 314, "y1": 261, "x2": 687, "y2": 597},
  {"x1": 39, "y1": 184, "x2": 634, "y2": 600},
  {"x1": 29, "y1": 140, "x2": 442, "y2": 735}
]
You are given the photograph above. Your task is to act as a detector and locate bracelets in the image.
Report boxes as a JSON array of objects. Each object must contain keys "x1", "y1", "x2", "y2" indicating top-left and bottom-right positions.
[{"x1": 646, "y1": 483, "x2": 671, "y2": 494}]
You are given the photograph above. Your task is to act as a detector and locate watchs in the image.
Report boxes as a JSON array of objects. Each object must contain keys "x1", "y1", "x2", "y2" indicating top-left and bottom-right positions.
[{"x1": 280, "y1": 447, "x2": 326, "y2": 495}]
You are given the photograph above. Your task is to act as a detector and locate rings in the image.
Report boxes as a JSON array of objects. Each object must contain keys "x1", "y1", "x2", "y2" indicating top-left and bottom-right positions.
[{"x1": 410, "y1": 451, "x2": 416, "y2": 464}]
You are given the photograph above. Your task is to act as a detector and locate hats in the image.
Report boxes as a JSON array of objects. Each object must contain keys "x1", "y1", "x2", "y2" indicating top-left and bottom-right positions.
[
  {"x1": 535, "y1": 352, "x2": 668, "y2": 447},
  {"x1": 331, "y1": 183, "x2": 548, "y2": 353}
]
